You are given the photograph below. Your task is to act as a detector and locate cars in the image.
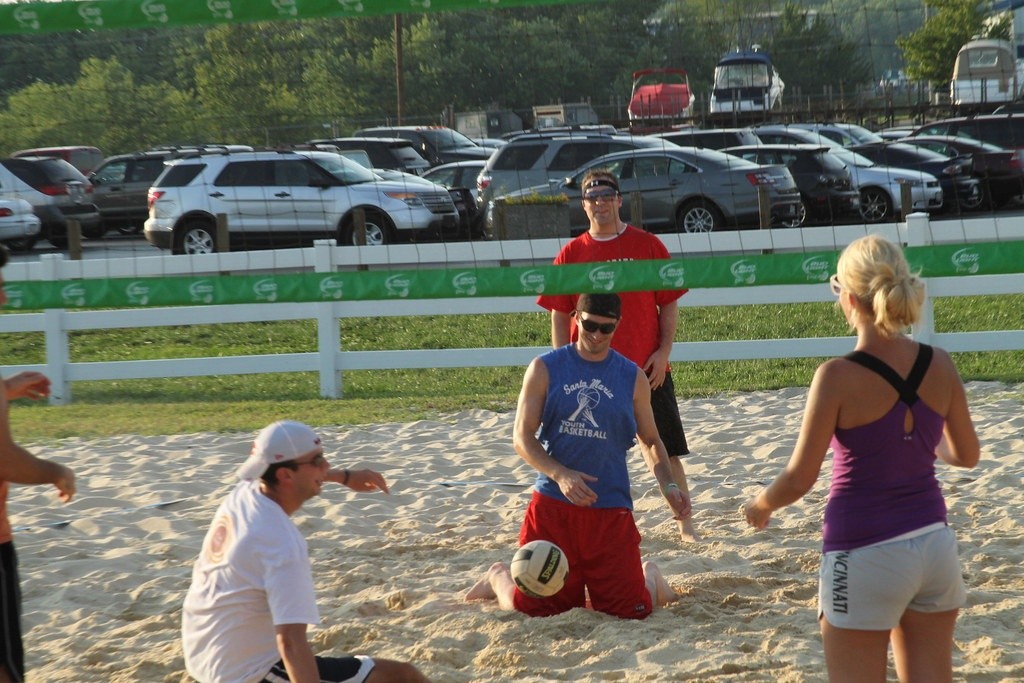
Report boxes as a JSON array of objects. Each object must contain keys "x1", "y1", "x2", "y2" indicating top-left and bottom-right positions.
[
  {"x1": 0, "y1": 196, "x2": 41, "y2": 245},
  {"x1": 0, "y1": 145, "x2": 110, "y2": 248},
  {"x1": 296, "y1": 110, "x2": 1024, "y2": 244}
]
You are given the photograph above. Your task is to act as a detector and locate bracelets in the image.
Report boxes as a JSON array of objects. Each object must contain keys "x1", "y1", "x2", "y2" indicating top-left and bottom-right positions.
[
  {"x1": 341, "y1": 468, "x2": 351, "y2": 487},
  {"x1": 663, "y1": 482, "x2": 678, "y2": 500}
]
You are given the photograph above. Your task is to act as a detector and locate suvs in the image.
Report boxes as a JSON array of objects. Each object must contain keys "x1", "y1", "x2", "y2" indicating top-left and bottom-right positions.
[{"x1": 143, "y1": 149, "x2": 460, "y2": 261}]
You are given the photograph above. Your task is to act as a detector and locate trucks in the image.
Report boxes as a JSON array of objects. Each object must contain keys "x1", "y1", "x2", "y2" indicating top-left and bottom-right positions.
[
  {"x1": 627, "y1": 68, "x2": 696, "y2": 123},
  {"x1": 453, "y1": 110, "x2": 525, "y2": 139},
  {"x1": 531, "y1": 103, "x2": 600, "y2": 129},
  {"x1": 946, "y1": 37, "x2": 1024, "y2": 102},
  {"x1": 710, "y1": 44, "x2": 785, "y2": 111}
]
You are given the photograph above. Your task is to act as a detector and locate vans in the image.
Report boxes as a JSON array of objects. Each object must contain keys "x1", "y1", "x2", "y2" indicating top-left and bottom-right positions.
[{"x1": 77, "y1": 145, "x2": 233, "y2": 242}]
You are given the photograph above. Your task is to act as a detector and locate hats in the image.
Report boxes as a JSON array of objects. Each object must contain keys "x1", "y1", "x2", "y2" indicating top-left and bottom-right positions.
[
  {"x1": 569, "y1": 293, "x2": 622, "y2": 320},
  {"x1": 235, "y1": 420, "x2": 322, "y2": 480}
]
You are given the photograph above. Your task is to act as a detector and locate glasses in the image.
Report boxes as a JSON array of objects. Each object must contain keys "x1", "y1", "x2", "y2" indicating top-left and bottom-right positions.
[
  {"x1": 830, "y1": 274, "x2": 849, "y2": 297},
  {"x1": 281, "y1": 454, "x2": 322, "y2": 467},
  {"x1": 579, "y1": 314, "x2": 619, "y2": 335},
  {"x1": 582, "y1": 189, "x2": 620, "y2": 203}
]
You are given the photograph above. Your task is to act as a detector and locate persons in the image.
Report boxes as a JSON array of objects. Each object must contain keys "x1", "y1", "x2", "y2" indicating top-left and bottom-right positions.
[
  {"x1": 181, "y1": 419, "x2": 430, "y2": 683},
  {"x1": 465, "y1": 293, "x2": 693, "y2": 622},
  {"x1": 0, "y1": 242, "x2": 77, "y2": 683},
  {"x1": 535, "y1": 168, "x2": 706, "y2": 546},
  {"x1": 737, "y1": 236, "x2": 980, "y2": 682}
]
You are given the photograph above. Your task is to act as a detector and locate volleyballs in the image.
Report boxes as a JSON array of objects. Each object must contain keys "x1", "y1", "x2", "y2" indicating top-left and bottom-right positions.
[{"x1": 510, "y1": 539, "x2": 569, "y2": 598}]
